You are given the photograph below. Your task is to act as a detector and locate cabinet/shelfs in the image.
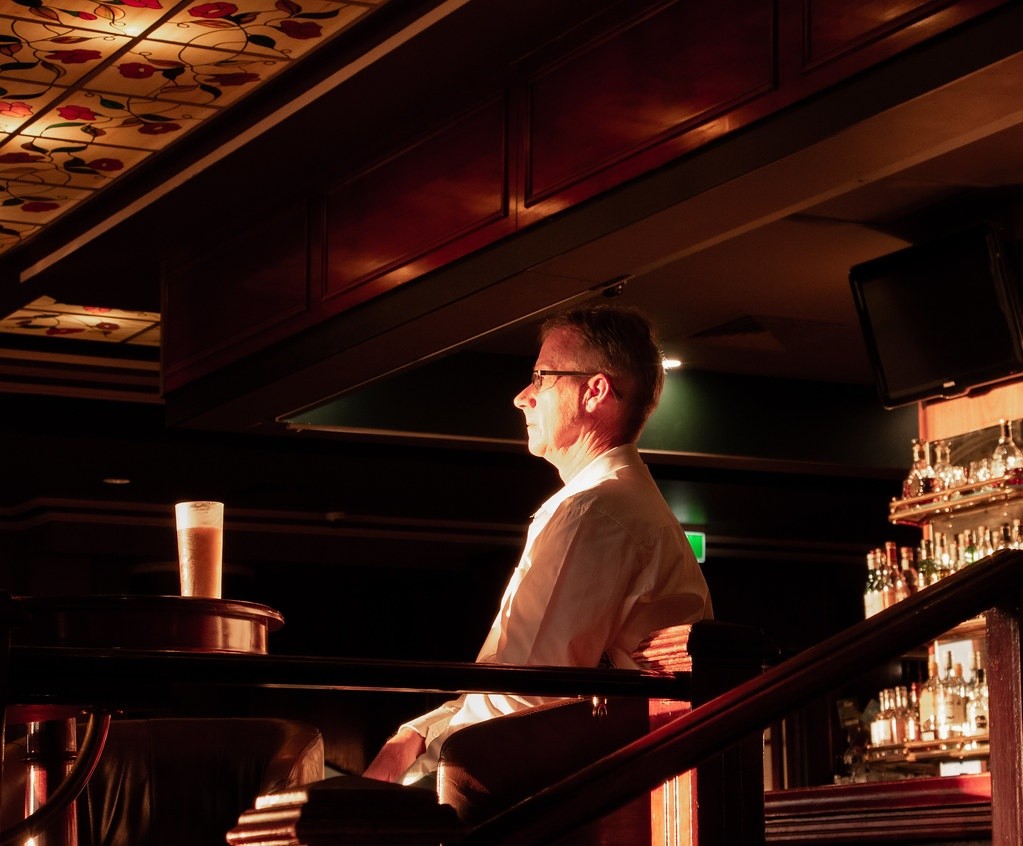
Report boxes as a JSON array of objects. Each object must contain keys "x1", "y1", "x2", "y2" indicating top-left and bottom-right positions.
[{"x1": 857, "y1": 474, "x2": 1023, "y2": 765}]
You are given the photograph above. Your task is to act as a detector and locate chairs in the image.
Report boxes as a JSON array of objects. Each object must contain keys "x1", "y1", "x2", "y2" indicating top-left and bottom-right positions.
[{"x1": 372, "y1": 686, "x2": 652, "y2": 846}]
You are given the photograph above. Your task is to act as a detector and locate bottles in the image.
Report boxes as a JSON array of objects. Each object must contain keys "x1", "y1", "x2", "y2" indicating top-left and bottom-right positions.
[
  {"x1": 863, "y1": 419, "x2": 1023, "y2": 749},
  {"x1": 176, "y1": 502, "x2": 222, "y2": 599}
]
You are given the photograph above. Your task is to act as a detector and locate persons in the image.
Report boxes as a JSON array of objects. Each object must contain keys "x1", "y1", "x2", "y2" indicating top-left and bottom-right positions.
[{"x1": 363, "y1": 307, "x2": 716, "y2": 786}]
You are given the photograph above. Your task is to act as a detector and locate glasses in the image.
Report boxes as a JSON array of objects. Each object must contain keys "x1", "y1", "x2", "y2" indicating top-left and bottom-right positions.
[{"x1": 531, "y1": 370, "x2": 622, "y2": 403}]
